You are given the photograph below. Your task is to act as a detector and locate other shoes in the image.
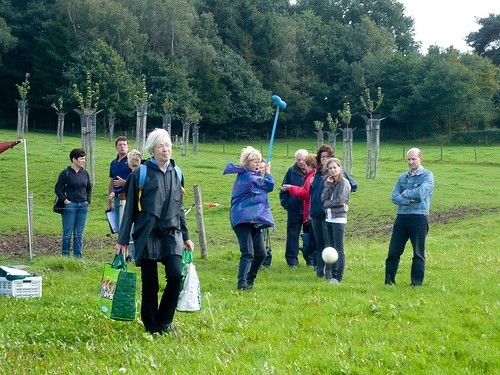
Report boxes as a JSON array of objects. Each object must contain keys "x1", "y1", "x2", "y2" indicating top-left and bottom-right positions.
[
  {"x1": 164, "y1": 324, "x2": 175, "y2": 335},
  {"x1": 329, "y1": 278, "x2": 340, "y2": 284}
]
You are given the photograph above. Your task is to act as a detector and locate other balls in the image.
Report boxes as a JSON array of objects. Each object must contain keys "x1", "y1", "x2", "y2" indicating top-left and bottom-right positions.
[{"x1": 322, "y1": 246, "x2": 339, "y2": 265}]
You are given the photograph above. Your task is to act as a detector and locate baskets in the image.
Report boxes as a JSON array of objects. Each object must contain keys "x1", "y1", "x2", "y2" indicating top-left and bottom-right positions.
[{"x1": 0, "y1": 276, "x2": 42, "y2": 299}]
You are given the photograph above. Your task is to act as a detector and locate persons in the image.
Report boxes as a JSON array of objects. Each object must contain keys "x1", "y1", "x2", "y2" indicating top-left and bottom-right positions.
[
  {"x1": 385, "y1": 147, "x2": 434, "y2": 287},
  {"x1": 115, "y1": 128, "x2": 194, "y2": 335},
  {"x1": 107, "y1": 137, "x2": 357, "y2": 292},
  {"x1": 54, "y1": 149, "x2": 92, "y2": 258}
]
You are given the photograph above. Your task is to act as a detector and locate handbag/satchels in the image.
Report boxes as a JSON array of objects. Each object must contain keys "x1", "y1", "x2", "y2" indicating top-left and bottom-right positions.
[
  {"x1": 100, "y1": 278, "x2": 117, "y2": 300},
  {"x1": 263, "y1": 245, "x2": 273, "y2": 267},
  {"x1": 176, "y1": 248, "x2": 202, "y2": 313},
  {"x1": 110, "y1": 262, "x2": 137, "y2": 321},
  {"x1": 52, "y1": 194, "x2": 65, "y2": 214}
]
